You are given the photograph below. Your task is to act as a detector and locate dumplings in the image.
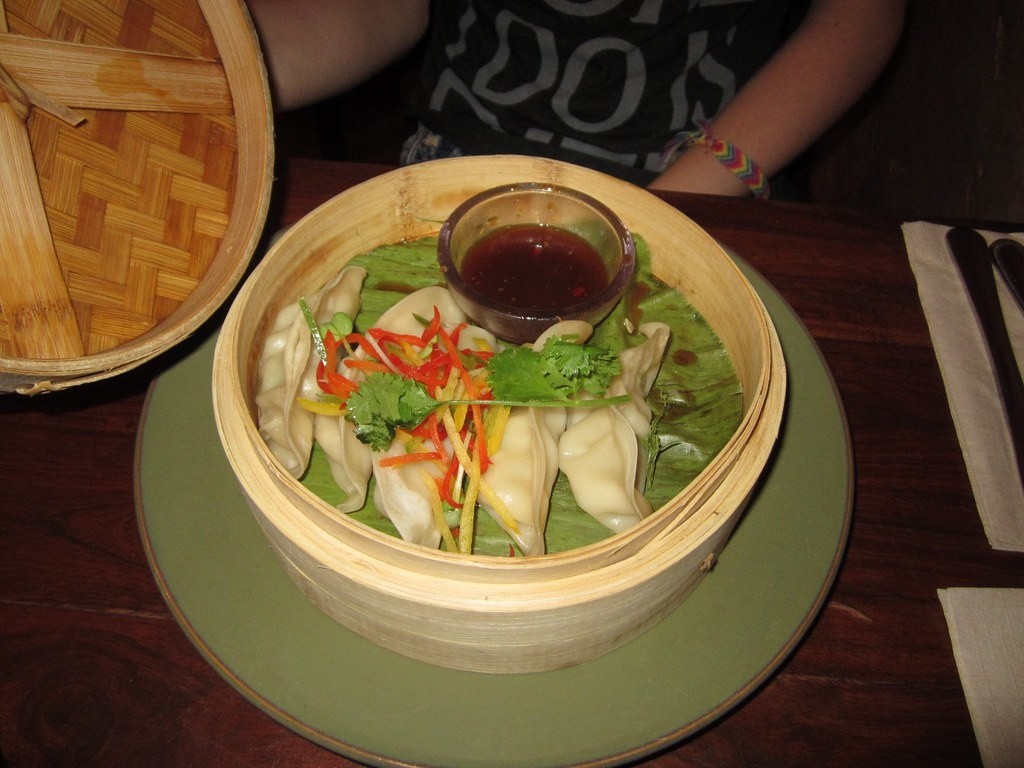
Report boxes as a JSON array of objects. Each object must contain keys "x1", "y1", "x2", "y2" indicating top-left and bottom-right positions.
[
  {"x1": 465, "y1": 319, "x2": 595, "y2": 559},
  {"x1": 368, "y1": 325, "x2": 498, "y2": 552},
  {"x1": 556, "y1": 322, "x2": 672, "y2": 532},
  {"x1": 253, "y1": 265, "x2": 368, "y2": 480},
  {"x1": 311, "y1": 284, "x2": 466, "y2": 515}
]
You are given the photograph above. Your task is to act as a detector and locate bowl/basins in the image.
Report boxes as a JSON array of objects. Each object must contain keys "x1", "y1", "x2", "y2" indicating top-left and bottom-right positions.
[{"x1": 439, "y1": 180, "x2": 634, "y2": 344}]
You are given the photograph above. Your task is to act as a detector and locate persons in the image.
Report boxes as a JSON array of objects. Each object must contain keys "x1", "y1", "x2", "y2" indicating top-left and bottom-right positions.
[{"x1": 243, "y1": 0, "x2": 1023, "y2": 235}]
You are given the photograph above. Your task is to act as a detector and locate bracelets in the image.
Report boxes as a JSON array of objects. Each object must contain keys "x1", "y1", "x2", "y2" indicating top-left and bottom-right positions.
[{"x1": 658, "y1": 110, "x2": 777, "y2": 203}]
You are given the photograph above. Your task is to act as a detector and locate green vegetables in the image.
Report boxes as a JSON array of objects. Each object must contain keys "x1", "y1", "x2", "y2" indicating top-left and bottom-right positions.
[{"x1": 337, "y1": 330, "x2": 632, "y2": 452}]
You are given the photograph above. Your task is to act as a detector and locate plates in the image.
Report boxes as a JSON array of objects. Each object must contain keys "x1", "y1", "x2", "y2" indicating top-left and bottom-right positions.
[{"x1": 129, "y1": 233, "x2": 855, "y2": 766}]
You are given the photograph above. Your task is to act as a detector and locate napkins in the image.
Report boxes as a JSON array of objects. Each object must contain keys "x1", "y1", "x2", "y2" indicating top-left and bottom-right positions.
[
  {"x1": 901, "y1": 222, "x2": 1024, "y2": 555},
  {"x1": 937, "y1": 587, "x2": 1023, "y2": 768}
]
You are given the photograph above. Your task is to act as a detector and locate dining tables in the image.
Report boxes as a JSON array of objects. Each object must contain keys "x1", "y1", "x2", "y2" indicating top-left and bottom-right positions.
[{"x1": 1, "y1": 154, "x2": 1024, "y2": 768}]
